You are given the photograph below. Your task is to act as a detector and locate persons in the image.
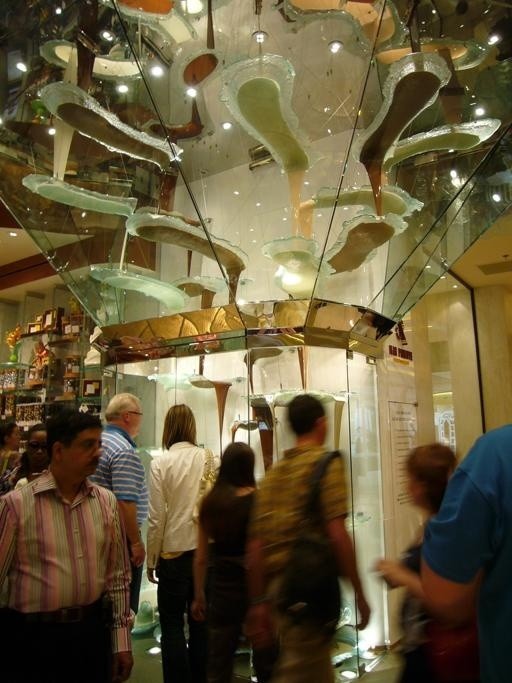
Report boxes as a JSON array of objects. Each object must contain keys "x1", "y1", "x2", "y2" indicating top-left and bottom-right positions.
[
  {"x1": 368, "y1": 442, "x2": 480, "y2": 683},
  {"x1": 14, "y1": 422, "x2": 51, "y2": 489},
  {"x1": 145, "y1": 403, "x2": 223, "y2": 683},
  {"x1": 90, "y1": 393, "x2": 146, "y2": 625},
  {"x1": 0, "y1": 411, "x2": 136, "y2": 681},
  {"x1": 244, "y1": 394, "x2": 370, "y2": 682},
  {"x1": 2, "y1": 418, "x2": 23, "y2": 489},
  {"x1": 192, "y1": 442, "x2": 279, "y2": 682},
  {"x1": 420, "y1": 422, "x2": 512, "y2": 683}
]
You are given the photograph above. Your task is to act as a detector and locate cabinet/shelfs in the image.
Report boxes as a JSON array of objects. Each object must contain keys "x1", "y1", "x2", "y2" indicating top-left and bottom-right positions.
[{"x1": 0, "y1": 306, "x2": 156, "y2": 445}]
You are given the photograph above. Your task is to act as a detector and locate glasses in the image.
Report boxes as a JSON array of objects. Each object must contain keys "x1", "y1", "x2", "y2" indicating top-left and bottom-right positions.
[
  {"x1": 128, "y1": 410, "x2": 146, "y2": 416},
  {"x1": 28, "y1": 441, "x2": 49, "y2": 450}
]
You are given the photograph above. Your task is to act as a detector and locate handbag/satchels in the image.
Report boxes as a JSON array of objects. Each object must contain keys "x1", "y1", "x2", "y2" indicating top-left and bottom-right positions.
[{"x1": 283, "y1": 537, "x2": 341, "y2": 632}]
